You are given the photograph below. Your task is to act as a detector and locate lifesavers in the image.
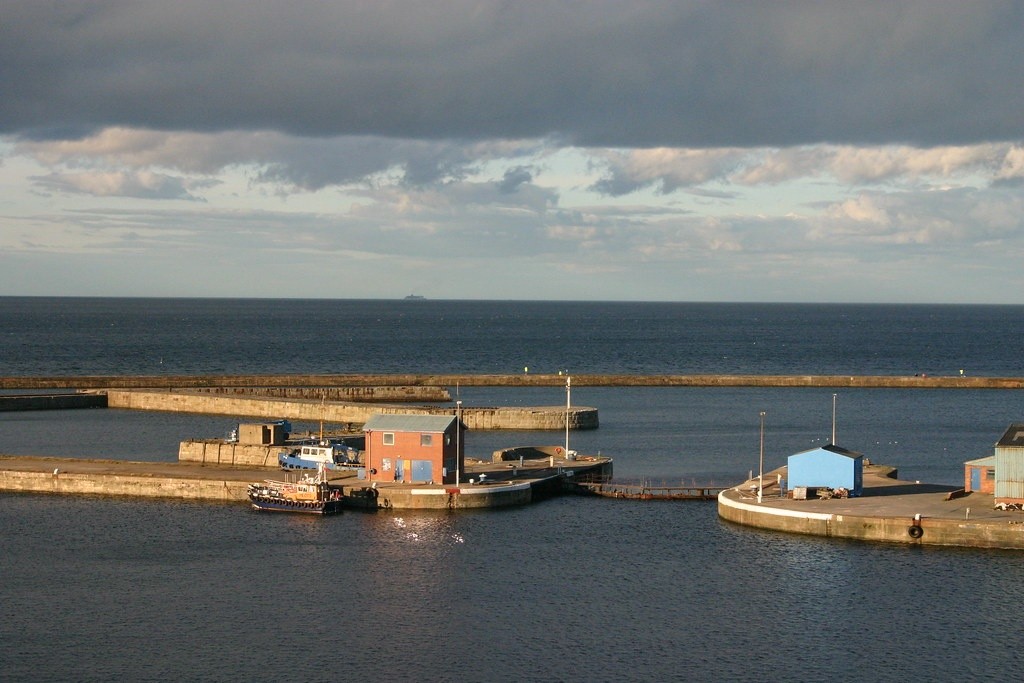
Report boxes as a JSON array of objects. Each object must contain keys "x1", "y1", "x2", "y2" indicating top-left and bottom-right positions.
[
  {"x1": 370, "y1": 468, "x2": 376, "y2": 474},
  {"x1": 283, "y1": 463, "x2": 287, "y2": 468},
  {"x1": 307, "y1": 503, "x2": 313, "y2": 510},
  {"x1": 908, "y1": 526, "x2": 923, "y2": 538},
  {"x1": 282, "y1": 500, "x2": 288, "y2": 506},
  {"x1": 295, "y1": 501, "x2": 300, "y2": 508},
  {"x1": 247, "y1": 490, "x2": 251, "y2": 495},
  {"x1": 288, "y1": 501, "x2": 293, "y2": 508},
  {"x1": 384, "y1": 499, "x2": 388, "y2": 507},
  {"x1": 264, "y1": 498, "x2": 270, "y2": 504},
  {"x1": 279, "y1": 460, "x2": 282, "y2": 465},
  {"x1": 249, "y1": 493, "x2": 253, "y2": 500},
  {"x1": 276, "y1": 499, "x2": 282, "y2": 505},
  {"x1": 270, "y1": 499, "x2": 275, "y2": 504},
  {"x1": 336, "y1": 491, "x2": 341, "y2": 498},
  {"x1": 289, "y1": 464, "x2": 293, "y2": 469},
  {"x1": 303, "y1": 467, "x2": 308, "y2": 469},
  {"x1": 301, "y1": 503, "x2": 306, "y2": 508},
  {"x1": 259, "y1": 497, "x2": 264, "y2": 503},
  {"x1": 314, "y1": 503, "x2": 319, "y2": 508},
  {"x1": 296, "y1": 465, "x2": 301, "y2": 469}
]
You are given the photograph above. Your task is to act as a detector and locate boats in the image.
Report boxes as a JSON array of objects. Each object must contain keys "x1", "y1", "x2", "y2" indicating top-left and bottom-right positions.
[
  {"x1": 248, "y1": 461, "x2": 355, "y2": 514},
  {"x1": 278, "y1": 443, "x2": 364, "y2": 471}
]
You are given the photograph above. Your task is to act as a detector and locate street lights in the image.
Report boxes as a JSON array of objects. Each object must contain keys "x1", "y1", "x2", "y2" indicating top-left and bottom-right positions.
[
  {"x1": 757, "y1": 411, "x2": 766, "y2": 505},
  {"x1": 565, "y1": 377, "x2": 571, "y2": 460},
  {"x1": 455, "y1": 400, "x2": 462, "y2": 489},
  {"x1": 833, "y1": 393, "x2": 836, "y2": 446}
]
